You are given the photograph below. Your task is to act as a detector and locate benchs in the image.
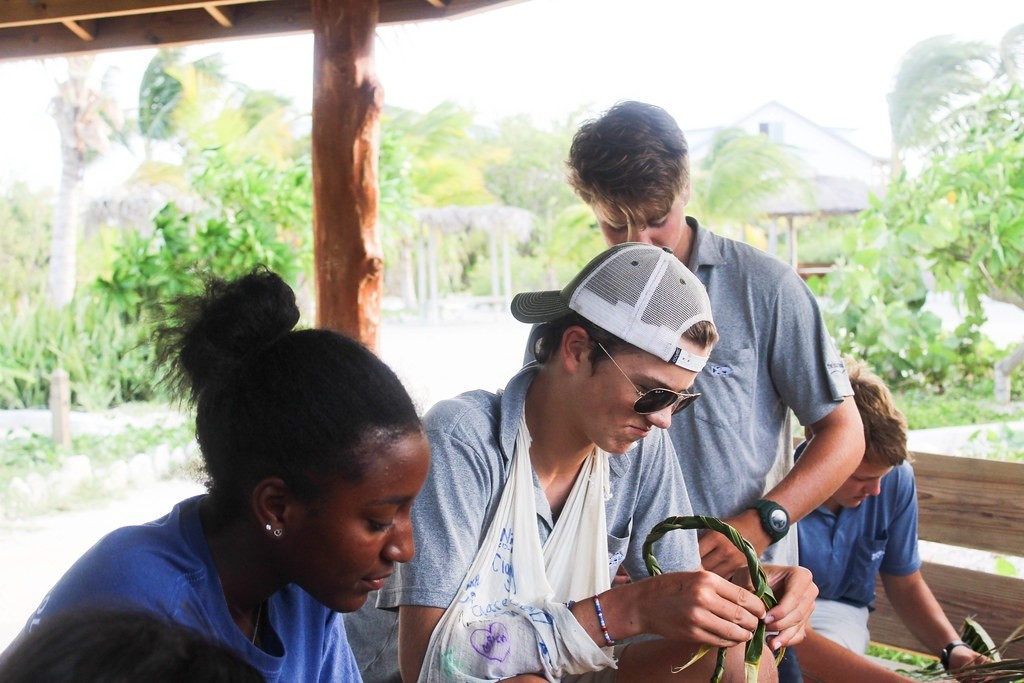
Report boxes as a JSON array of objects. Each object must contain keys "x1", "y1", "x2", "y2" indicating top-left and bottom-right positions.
[{"x1": 788, "y1": 437, "x2": 1024, "y2": 683}]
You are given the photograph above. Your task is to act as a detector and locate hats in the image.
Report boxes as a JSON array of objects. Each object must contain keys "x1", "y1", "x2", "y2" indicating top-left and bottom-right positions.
[{"x1": 509, "y1": 239, "x2": 720, "y2": 375}]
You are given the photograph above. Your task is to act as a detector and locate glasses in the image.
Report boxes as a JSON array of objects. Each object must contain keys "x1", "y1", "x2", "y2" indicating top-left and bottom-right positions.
[{"x1": 590, "y1": 338, "x2": 700, "y2": 423}]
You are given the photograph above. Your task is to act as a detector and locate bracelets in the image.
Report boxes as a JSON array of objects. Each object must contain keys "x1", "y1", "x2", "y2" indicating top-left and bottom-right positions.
[
  {"x1": 937, "y1": 640, "x2": 971, "y2": 677},
  {"x1": 590, "y1": 592, "x2": 616, "y2": 648}
]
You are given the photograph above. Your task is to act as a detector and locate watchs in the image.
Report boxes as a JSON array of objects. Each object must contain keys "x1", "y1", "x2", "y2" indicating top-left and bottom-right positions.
[{"x1": 747, "y1": 498, "x2": 790, "y2": 546}]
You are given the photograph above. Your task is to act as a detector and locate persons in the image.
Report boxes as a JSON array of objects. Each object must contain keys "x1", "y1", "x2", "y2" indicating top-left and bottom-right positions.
[
  {"x1": 519, "y1": 96, "x2": 864, "y2": 605},
  {"x1": 772, "y1": 370, "x2": 1001, "y2": 683},
  {"x1": 340, "y1": 239, "x2": 821, "y2": 683},
  {"x1": 2, "y1": 262, "x2": 433, "y2": 682}
]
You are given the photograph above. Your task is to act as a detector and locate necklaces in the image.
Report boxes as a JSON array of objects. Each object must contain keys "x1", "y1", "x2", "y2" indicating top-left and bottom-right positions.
[{"x1": 248, "y1": 601, "x2": 264, "y2": 648}]
